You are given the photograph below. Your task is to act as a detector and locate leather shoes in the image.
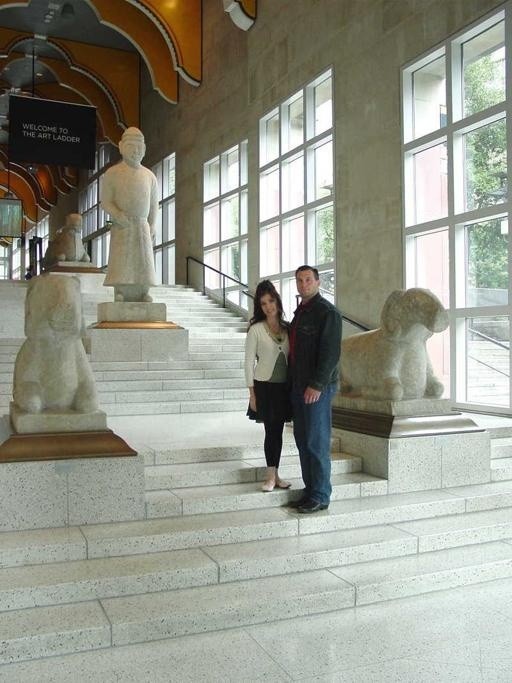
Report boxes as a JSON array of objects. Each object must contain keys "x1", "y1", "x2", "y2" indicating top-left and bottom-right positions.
[
  {"x1": 297, "y1": 500, "x2": 328, "y2": 513},
  {"x1": 288, "y1": 497, "x2": 309, "y2": 507},
  {"x1": 262, "y1": 479, "x2": 275, "y2": 492},
  {"x1": 275, "y1": 480, "x2": 291, "y2": 489}
]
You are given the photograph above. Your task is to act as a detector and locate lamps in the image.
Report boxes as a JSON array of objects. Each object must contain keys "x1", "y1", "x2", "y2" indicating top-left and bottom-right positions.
[
  {"x1": 26, "y1": 163, "x2": 39, "y2": 175},
  {"x1": 60, "y1": 0, "x2": 75, "y2": 20},
  {"x1": 0, "y1": 159, "x2": 23, "y2": 238}
]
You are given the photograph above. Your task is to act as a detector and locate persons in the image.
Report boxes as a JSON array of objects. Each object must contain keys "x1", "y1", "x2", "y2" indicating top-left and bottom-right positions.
[
  {"x1": 98, "y1": 127, "x2": 160, "y2": 303},
  {"x1": 245, "y1": 280, "x2": 293, "y2": 492},
  {"x1": 289, "y1": 265, "x2": 342, "y2": 515}
]
